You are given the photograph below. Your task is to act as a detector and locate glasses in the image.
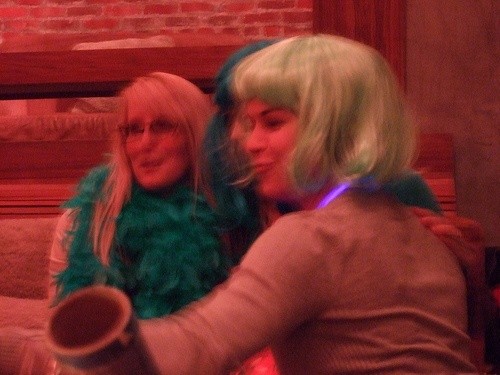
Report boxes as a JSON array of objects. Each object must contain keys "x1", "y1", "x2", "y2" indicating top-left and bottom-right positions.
[{"x1": 114, "y1": 119, "x2": 180, "y2": 143}]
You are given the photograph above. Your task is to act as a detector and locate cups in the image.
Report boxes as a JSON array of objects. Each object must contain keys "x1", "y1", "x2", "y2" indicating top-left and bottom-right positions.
[{"x1": 44, "y1": 284, "x2": 162, "y2": 374}]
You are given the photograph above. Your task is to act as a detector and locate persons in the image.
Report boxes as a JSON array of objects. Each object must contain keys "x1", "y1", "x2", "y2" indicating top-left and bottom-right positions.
[
  {"x1": 199, "y1": 35, "x2": 488, "y2": 325},
  {"x1": 1, "y1": 32, "x2": 488, "y2": 375},
  {"x1": 44, "y1": 69, "x2": 279, "y2": 375}
]
